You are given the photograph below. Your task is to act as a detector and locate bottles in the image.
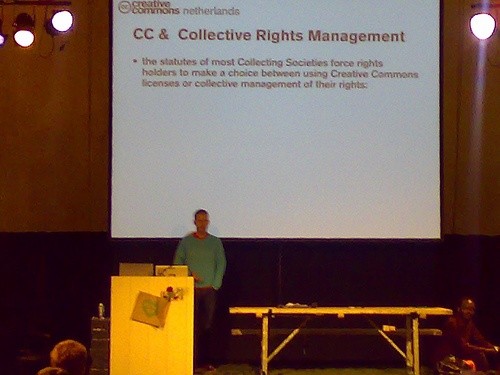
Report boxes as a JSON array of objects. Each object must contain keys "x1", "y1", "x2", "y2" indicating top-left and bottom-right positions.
[{"x1": 98, "y1": 302, "x2": 103, "y2": 318}]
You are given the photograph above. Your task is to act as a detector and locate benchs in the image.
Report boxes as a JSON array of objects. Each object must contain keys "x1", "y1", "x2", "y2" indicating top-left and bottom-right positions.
[{"x1": 232, "y1": 329, "x2": 441, "y2": 336}]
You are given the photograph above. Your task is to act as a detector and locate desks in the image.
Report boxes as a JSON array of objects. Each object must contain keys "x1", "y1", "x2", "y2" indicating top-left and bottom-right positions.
[
  {"x1": 229, "y1": 308, "x2": 453, "y2": 375},
  {"x1": 110, "y1": 276, "x2": 194, "y2": 375}
]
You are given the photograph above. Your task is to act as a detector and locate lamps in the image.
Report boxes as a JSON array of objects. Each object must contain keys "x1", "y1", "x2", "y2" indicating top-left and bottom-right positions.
[
  {"x1": 43, "y1": 10, "x2": 75, "y2": 39},
  {"x1": 14, "y1": 12, "x2": 35, "y2": 47}
]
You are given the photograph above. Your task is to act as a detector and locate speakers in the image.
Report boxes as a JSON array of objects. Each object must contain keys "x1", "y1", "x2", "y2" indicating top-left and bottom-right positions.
[{"x1": 88, "y1": 317, "x2": 110, "y2": 375}]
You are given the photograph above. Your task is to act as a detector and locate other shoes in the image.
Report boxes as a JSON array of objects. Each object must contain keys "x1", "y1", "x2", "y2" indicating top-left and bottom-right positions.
[{"x1": 203, "y1": 363, "x2": 216, "y2": 371}]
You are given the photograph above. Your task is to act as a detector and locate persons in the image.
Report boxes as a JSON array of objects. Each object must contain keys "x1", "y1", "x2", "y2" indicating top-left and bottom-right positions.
[
  {"x1": 36, "y1": 339, "x2": 89, "y2": 375},
  {"x1": 430, "y1": 297, "x2": 500, "y2": 375},
  {"x1": 174, "y1": 209, "x2": 228, "y2": 372}
]
